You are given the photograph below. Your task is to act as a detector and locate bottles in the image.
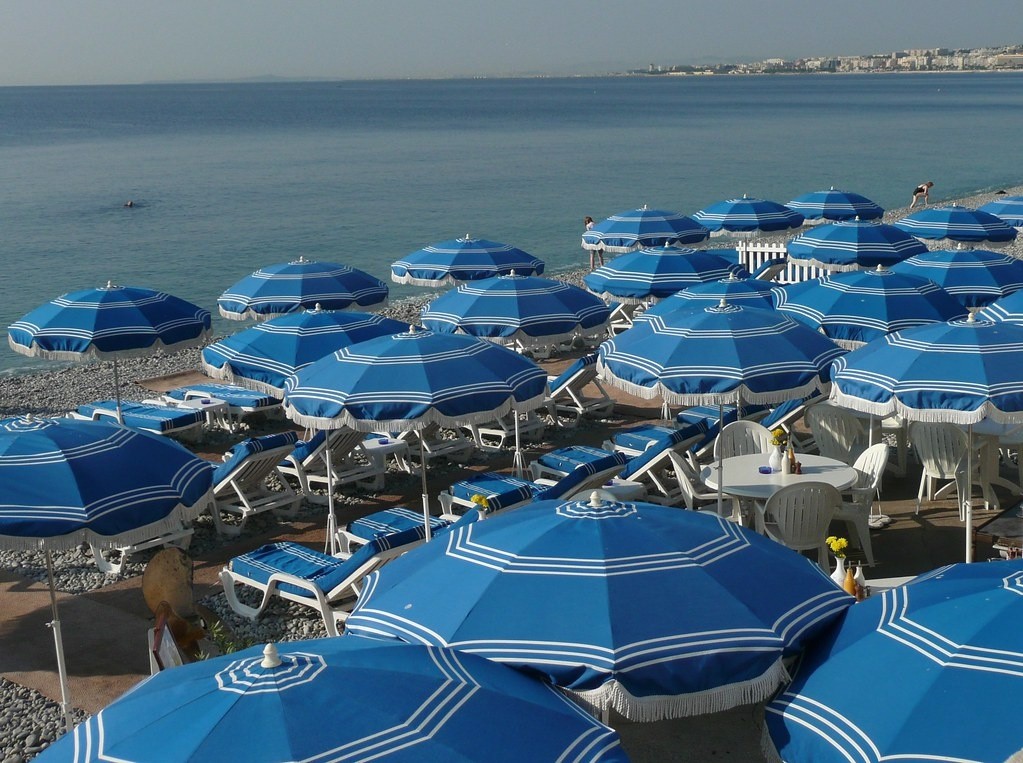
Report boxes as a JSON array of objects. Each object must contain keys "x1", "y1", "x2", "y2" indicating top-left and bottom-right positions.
[
  {"x1": 853, "y1": 565, "x2": 865, "y2": 588},
  {"x1": 782, "y1": 450, "x2": 790, "y2": 474},
  {"x1": 844, "y1": 568, "x2": 856, "y2": 595},
  {"x1": 1007, "y1": 546, "x2": 1018, "y2": 561},
  {"x1": 788, "y1": 447, "x2": 795, "y2": 466},
  {"x1": 785, "y1": 432, "x2": 794, "y2": 453}
]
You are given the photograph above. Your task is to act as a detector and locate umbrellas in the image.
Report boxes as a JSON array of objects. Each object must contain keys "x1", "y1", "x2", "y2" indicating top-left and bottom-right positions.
[{"x1": 0, "y1": 186, "x2": 1023, "y2": 763}]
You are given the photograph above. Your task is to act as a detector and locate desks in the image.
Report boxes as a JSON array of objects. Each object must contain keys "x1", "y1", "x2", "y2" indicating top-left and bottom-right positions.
[
  {"x1": 699, "y1": 452, "x2": 858, "y2": 500},
  {"x1": 809, "y1": 397, "x2": 897, "y2": 443},
  {"x1": 882, "y1": 414, "x2": 1023, "y2": 497}
]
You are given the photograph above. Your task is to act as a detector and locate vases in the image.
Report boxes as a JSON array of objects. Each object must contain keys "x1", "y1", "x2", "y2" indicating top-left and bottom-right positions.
[
  {"x1": 787, "y1": 448, "x2": 795, "y2": 474},
  {"x1": 829, "y1": 557, "x2": 846, "y2": 588},
  {"x1": 777, "y1": 446, "x2": 785, "y2": 470},
  {"x1": 476, "y1": 510, "x2": 487, "y2": 522}
]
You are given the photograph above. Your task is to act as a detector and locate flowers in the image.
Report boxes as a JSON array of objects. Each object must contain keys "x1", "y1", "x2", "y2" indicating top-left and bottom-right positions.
[
  {"x1": 770, "y1": 429, "x2": 790, "y2": 445},
  {"x1": 824, "y1": 536, "x2": 847, "y2": 558},
  {"x1": 472, "y1": 494, "x2": 488, "y2": 510}
]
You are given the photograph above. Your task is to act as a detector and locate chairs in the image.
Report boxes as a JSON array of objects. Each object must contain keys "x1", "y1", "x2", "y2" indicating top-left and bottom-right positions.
[{"x1": 65, "y1": 258, "x2": 1023, "y2": 637}]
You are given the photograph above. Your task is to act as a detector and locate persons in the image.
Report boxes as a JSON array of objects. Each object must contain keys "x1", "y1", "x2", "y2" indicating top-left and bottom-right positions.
[
  {"x1": 910, "y1": 182, "x2": 933, "y2": 208},
  {"x1": 584, "y1": 216, "x2": 603, "y2": 272}
]
